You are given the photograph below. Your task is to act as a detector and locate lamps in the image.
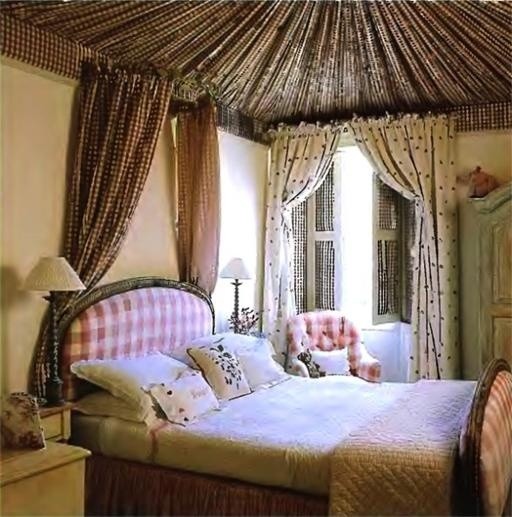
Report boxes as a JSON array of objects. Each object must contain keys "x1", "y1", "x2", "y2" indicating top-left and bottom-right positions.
[
  {"x1": 219, "y1": 259, "x2": 251, "y2": 333},
  {"x1": 25, "y1": 254, "x2": 88, "y2": 410}
]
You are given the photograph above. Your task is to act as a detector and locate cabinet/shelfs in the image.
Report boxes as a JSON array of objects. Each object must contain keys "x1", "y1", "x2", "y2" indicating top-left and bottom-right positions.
[{"x1": 469, "y1": 176, "x2": 512, "y2": 378}]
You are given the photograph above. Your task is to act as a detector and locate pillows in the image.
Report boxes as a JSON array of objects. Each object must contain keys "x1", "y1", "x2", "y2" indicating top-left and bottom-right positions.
[
  {"x1": 70, "y1": 350, "x2": 192, "y2": 406},
  {"x1": 73, "y1": 392, "x2": 140, "y2": 423},
  {"x1": 186, "y1": 344, "x2": 250, "y2": 404},
  {"x1": 145, "y1": 367, "x2": 219, "y2": 427},
  {"x1": 163, "y1": 333, "x2": 261, "y2": 369},
  {"x1": 235, "y1": 342, "x2": 281, "y2": 389},
  {"x1": 309, "y1": 349, "x2": 353, "y2": 375}
]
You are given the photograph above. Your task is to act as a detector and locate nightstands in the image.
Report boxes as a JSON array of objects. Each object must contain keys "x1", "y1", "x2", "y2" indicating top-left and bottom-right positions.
[{"x1": 0, "y1": 442, "x2": 93, "y2": 517}]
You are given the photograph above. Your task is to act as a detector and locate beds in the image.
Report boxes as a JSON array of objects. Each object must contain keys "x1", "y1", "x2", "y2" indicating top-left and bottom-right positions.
[{"x1": 28, "y1": 275, "x2": 512, "y2": 517}]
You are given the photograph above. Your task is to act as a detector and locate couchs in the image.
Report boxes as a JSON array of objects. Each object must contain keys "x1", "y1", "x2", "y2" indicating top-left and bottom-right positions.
[{"x1": 285, "y1": 307, "x2": 381, "y2": 383}]
